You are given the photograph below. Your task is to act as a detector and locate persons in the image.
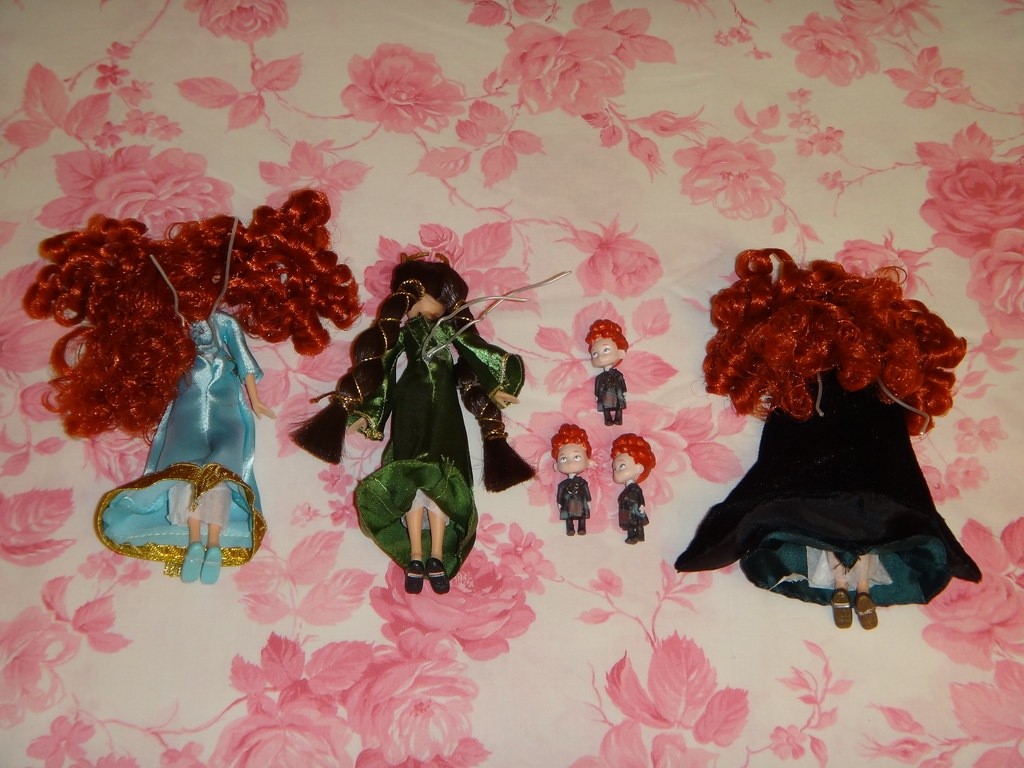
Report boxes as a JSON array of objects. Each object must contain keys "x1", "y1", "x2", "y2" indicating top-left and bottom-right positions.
[
  {"x1": 552, "y1": 423, "x2": 593, "y2": 536},
  {"x1": 676, "y1": 249, "x2": 983, "y2": 630},
  {"x1": 289, "y1": 254, "x2": 537, "y2": 596},
  {"x1": 611, "y1": 432, "x2": 655, "y2": 544},
  {"x1": 586, "y1": 321, "x2": 629, "y2": 428},
  {"x1": 20, "y1": 192, "x2": 362, "y2": 587}
]
